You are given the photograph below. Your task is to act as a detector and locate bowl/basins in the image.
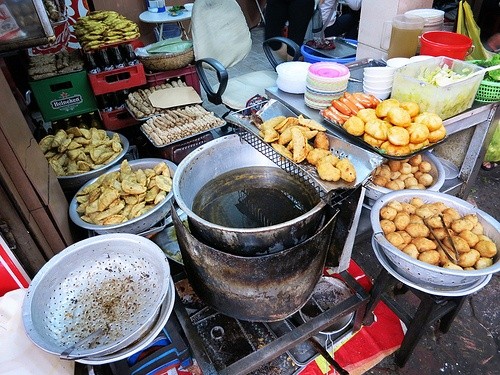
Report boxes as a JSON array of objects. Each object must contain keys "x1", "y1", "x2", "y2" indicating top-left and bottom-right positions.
[
  {"x1": 370, "y1": 189, "x2": 500, "y2": 285},
  {"x1": 371, "y1": 232, "x2": 493, "y2": 296},
  {"x1": 275, "y1": 60, "x2": 313, "y2": 96},
  {"x1": 302, "y1": 277, "x2": 355, "y2": 336},
  {"x1": 184, "y1": 3, "x2": 194, "y2": 10},
  {"x1": 59, "y1": 128, "x2": 128, "y2": 190},
  {"x1": 365, "y1": 150, "x2": 445, "y2": 199},
  {"x1": 74, "y1": 274, "x2": 175, "y2": 365},
  {"x1": 171, "y1": 133, "x2": 327, "y2": 255},
  {"x1": 68, "y1": 158, "x2": 176, "y2": 234},
  {"x1": 361, "y1": 54, "x2": 435, "y2": 102},
  {"x1": 21, "y1": 232, "x2": 172, "y2": 359}
]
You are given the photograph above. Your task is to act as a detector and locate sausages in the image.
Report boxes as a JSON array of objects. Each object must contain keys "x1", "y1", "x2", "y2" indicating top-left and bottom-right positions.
[{"x1": 321, "y1": 92, "x2": 382, "y2": 127}]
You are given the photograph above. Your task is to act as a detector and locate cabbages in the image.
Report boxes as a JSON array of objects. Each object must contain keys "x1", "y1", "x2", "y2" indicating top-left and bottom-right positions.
[{"x1": 395, "y1": 64, "x2": 476, "y2": 119}]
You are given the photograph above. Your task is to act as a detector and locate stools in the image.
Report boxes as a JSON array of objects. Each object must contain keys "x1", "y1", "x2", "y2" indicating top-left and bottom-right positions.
[{"x1": 364, "y1": 267, "x2": 468, "y2": 368}]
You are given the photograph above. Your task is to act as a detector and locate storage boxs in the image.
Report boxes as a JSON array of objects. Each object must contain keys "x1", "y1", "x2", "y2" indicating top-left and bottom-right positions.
[
  {"x1": 391, "y1": 56, "x2": 486, "y2": 121},
  {"x1": 29, "y1": 35, "x2": 201, "y2": 130}
]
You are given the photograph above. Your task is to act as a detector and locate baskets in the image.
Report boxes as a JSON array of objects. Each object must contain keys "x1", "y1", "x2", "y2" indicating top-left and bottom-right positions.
[{"x1": 462, "y1": 60, "x2": 500, "y2": 103}]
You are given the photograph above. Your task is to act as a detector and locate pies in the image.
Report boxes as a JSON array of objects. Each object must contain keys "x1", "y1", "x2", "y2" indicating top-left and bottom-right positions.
[
  {"x1": 343, "y1": 99, "x2": 445, "y2": 156},
  {"x1": 76, "y1": 159, "x2": 172, "y2": 227},
  {"x1": 39, "y1": 127, "x2": 122, "y2": 176},
  {"x1": 73, "y1": 10, "x2": 140, "y2": 48},
  {"x1": 258, "y1": 114, "x2": 329, "y2": 163}
]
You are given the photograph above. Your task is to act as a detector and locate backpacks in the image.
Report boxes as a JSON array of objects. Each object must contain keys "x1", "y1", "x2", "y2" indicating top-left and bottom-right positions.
[{"x1": 305, "y1": 8, "x2": 326, "y2": 42}]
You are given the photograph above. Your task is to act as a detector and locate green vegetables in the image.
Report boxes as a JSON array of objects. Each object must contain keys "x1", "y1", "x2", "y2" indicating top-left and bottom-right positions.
[{"x1": 471, "y1": 54, "x2": 500, "y2": 101}]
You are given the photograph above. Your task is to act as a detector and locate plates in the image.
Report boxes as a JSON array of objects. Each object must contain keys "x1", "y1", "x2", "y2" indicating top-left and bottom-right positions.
[{"x1": 304, "y1": 62, "x2": 351, "y2": 110}]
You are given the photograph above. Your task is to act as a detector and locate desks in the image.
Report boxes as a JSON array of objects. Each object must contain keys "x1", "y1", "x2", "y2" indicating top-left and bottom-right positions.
[{"x1": 138, "y1": 6, "x2": 192, "y2": 42}]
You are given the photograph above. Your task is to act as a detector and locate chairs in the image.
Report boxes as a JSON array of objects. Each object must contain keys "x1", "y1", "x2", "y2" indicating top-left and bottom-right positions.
[{"x1": 191, "y1": 0, "x2": 301, "y2": 133}]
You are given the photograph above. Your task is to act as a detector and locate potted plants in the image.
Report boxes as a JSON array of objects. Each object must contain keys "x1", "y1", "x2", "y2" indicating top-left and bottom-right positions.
[{"x1": 168, "y1": 5, "x2": 181, "y2": 15}]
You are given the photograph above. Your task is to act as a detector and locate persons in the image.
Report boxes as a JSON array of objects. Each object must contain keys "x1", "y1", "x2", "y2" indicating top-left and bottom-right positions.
[
  {"x1": 319, "y1": 0, "x2": 362, "y2": 39},
  {"x1": 264, "y1": 0, "x2": 315, "y2": 62}
]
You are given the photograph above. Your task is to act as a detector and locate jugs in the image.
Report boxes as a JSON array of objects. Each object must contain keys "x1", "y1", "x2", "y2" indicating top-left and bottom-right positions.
[{"x1": 380, "y1": 12, "x2": 426, "y2": 62}]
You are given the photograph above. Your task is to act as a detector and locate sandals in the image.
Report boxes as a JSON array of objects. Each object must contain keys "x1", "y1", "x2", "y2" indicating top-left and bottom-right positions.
[{"x1": 481, "y1": 161, "x2": 500, "y2": 170}]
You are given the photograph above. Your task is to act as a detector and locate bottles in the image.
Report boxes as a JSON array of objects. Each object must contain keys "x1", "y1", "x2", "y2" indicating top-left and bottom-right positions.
[{"x1": 35, "y1": 43, "x2": 186, "y2": 143}]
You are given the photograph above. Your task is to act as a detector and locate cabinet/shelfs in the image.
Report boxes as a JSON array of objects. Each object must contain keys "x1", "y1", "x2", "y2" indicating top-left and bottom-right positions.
[{"x1": 265, "y1": 68, "x2": 500, "y2": 246}]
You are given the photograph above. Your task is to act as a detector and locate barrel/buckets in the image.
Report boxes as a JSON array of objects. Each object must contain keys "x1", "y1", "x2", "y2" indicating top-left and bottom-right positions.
[
  {"x1": 417, "y1": 29, "x2": 474, "y2": 60},
  {"x1": 405, "y1": 8, "x2": 446, "y2": 54},
  {"x1": 301, "y1": 38, "x2": 359, "y2": 65}
]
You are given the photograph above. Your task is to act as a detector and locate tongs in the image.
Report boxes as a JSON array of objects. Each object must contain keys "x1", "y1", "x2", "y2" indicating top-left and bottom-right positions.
[
  {"x1": 423, "y1": 215, "x2": 463, "y2": 263},
  {"x1": 59, "y1": 327, "x2": 104, "y2": 360}
]
